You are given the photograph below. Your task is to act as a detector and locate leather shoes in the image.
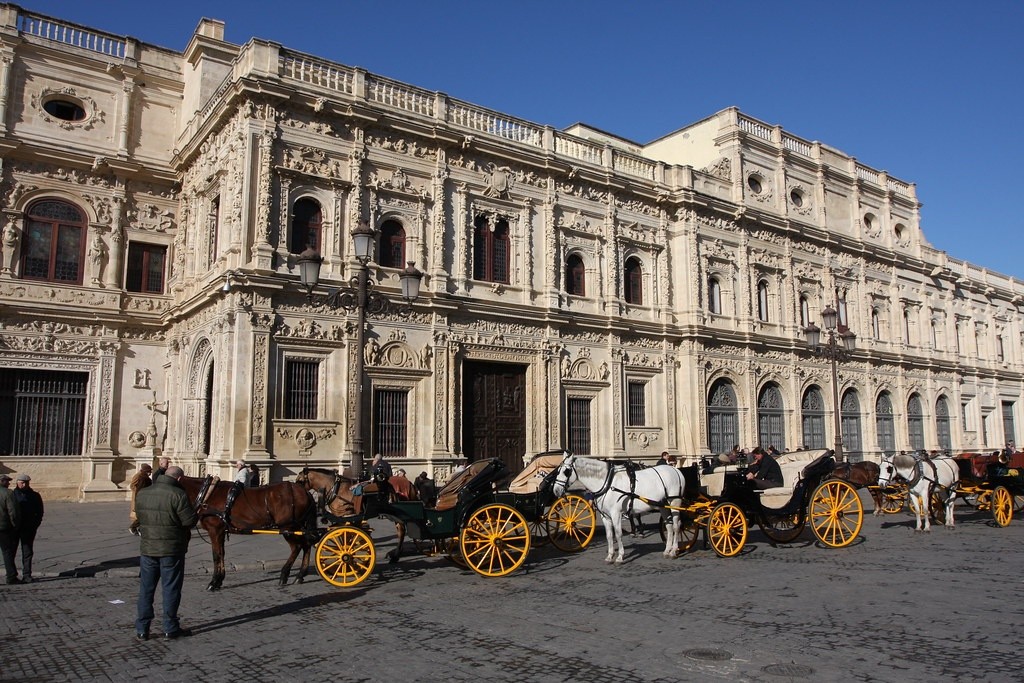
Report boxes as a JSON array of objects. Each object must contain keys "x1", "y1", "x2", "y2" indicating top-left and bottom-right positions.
[
  {"x1": 137, "y1": 633, "x2": 148, "y2": 641},
  {"x1": 166, "y1": 621, "x2": 179, "y2": 637}
]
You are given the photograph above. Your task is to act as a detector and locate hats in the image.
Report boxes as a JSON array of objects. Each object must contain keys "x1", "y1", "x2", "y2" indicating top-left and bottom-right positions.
[
  {"x1": 0, "y1": 475, "x2": 13, "y2": 480},
  {"x1": 17, "y1": 475, "x2": 31, "y2": 481}
]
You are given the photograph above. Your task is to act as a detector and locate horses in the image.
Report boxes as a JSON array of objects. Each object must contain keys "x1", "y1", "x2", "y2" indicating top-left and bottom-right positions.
[
  {"x1": 295, "y1": 467, "x2": 417, "y2": 563},
  {"x1": 878, "y1": 453, "x2": 960, "y2": 534},
  {"x1": 553, "y1": 451, "x2": 686, "y2": 566},
  {"x1": 153, "y1": 467, "x2": 316, "y2": 592},
  {"x1": 826, "y1": 461, "x2": 885, "y2": 517}
]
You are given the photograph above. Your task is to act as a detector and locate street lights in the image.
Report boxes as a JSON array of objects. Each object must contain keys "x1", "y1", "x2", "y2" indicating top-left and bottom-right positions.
[
  {"x1": 803, "y1": 304, "x2": 856, "y2": 465},
  {"x1": 294, "y1": 217, "x2": 426, "y2": 484}
]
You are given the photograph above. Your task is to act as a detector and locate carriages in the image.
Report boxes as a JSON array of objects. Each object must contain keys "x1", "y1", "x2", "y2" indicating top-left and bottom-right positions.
[
  {"x1": 361, "y1": 444, "x2": 948, "y2": 564},
  {"x1": 184, "y1": 456, "x2": 532, "y2": 590},
  {"x1": 877, "y1": 447, "x2": 1024, "y2": 533}
]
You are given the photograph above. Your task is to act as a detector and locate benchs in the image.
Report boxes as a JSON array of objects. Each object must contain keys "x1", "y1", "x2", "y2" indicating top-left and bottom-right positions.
[
  {"x1": 527, "y1": 472, "x2": 550, "y2": 492},
  {"x1": 758, "y1": 460, "x2": 812, "y2": 496},
  {"x1": 436, "y1": 487, "x2": 465, "y2": 510},
  {"x1": 338, "y1": 483, "x2": 364, "y2": 522}
]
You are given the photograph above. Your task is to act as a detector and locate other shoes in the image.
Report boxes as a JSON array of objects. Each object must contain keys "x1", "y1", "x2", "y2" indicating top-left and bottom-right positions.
[
  {"x1": 22, "y1": 576, "x2": 34, "y2": 582},
  {"x1": 6, "y1": 577, "x2": 24, "y2": 585}
]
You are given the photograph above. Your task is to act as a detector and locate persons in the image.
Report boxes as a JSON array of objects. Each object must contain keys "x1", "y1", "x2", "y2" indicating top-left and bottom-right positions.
[
  {"x1": 135, "y1": 466, "x2": 199, "y2": 641},
  {"x1": 0, "y1": 475, "x2": 44, "y2": 585},
  {"x1": 901, "y1": 450, "x2": 938, "y2": 461},
  {"x1": 1006, "y1": 440, "x2": 1024, "y2": 454},
  {"x1": 743, "y1": 446, "x2": 784, "y2": 496},
  {"x1": 1, "y1": 222, "x2": 20, "y2": 273},
  {"x1": 830, "y1": 450, "x2": 836, "y2": 462},
  {"x1": 418, "y1": 342, "x2": 432, "y2": 368},
  {"x1": 728, "y1": 445, "x2": 809, "y2": 464},
  {"x1": 234, "y1": 459, "x2": 260, "y2": 487},
  {"x1": 656, "y1": 452, "x2": 677, "y2": 467},
  {"x1": 365, "y1": 338, "x2": 380, "y2": 366},
  {"x1": 128, "y1": 459, "x2": 169, "y2": 537},
  {"x1": 600, "y1": 359, "x2": 610, "y2": 380},
  {"x1": 561, "y1": 355, "x2": 572, "y2": 379},
  {"x1": 366, "y1": 454, "x2": 429, "y2": 501},
  {"x1": 91, "y1": 235, "x2": 104, "y2": 283}
]
